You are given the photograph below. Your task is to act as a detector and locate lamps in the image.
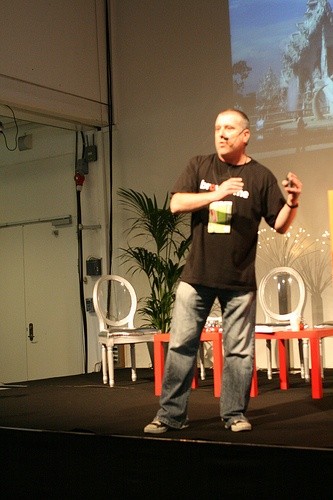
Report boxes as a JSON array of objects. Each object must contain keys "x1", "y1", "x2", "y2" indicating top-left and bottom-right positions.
[{"x1": 18, "y1": 134, "x2": 32, "y2": 151}]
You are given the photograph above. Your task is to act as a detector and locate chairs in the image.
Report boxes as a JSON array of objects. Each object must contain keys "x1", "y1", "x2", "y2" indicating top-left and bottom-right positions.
[
  {"x1": 259, "y1": 267, "x2": 324, "y2": 382},
  {"x1": 93, "y1": 275, "x2": 161, "y2": 387}
]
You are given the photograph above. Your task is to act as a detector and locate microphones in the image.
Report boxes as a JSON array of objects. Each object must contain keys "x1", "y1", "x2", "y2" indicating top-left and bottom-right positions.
[{"x1": 225, "y1": 129, "x2": 245, "y2": 140}]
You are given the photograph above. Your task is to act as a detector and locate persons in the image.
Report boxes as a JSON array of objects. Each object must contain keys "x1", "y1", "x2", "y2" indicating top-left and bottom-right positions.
[{"x1": 145, "y1": 108, "x2": 302, "y2": 434}]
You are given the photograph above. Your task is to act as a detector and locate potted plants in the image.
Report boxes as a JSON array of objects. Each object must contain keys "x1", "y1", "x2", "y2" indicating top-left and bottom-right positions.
[{"x1": 115, "y1": 184, "x2": 191, "y2": 367}]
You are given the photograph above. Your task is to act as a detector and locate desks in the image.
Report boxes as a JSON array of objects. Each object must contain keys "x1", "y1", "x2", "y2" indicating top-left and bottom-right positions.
[{"x1": 154, "y1": 327, "x2": 333, "y2": 400}]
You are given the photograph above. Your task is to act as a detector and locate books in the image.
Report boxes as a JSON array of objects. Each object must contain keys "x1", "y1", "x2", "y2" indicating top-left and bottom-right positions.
[
  {"x1": 314, "y1": 321, "x2": 333, "y2": 329},
  {"x1": 255, "y1": 324, "x2": 291, "y2": 334}
]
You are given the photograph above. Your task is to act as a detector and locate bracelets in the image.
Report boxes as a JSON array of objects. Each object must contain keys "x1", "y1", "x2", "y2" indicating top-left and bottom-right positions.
[{"x1": 287, "y1": 204, "x2": 299, "y2": 208}]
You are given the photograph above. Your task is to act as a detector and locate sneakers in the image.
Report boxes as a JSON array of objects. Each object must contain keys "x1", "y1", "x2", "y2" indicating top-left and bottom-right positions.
[
  {"x1": 225, "y1": 418, "x2": 251, "y2": 432},
  {"x1": 144, "y1": 418, "x2": 189, "y2": 433}
]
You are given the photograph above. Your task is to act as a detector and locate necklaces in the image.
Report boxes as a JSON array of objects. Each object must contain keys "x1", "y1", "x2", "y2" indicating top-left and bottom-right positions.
[{"x1": 216, "y1": 155, "x2": 247, "y2": 177}]
